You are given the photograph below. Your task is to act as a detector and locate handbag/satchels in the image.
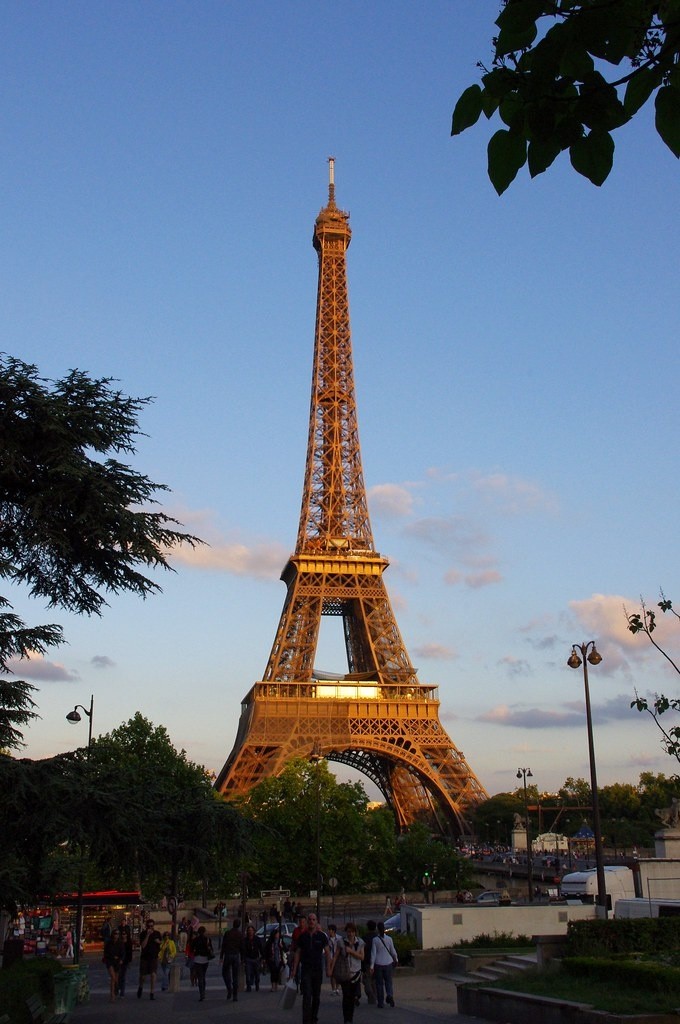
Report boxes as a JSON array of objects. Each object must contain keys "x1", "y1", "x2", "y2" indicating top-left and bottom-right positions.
[
  {"x1": 279, "y1": 975, "x2": 298, "y2": 1010},
  {"x1": 101, "y1": 957, "x2": 106, "y2": 963},
  {"x1": 332, "y1": 938, "x2": 350, "y2": 979},
  {"x1": 393, "y1": 958, "x2": 397, "y2": 968},
  {"x1": 167, "y1": 957, "x2": 174, "y2": 964}
]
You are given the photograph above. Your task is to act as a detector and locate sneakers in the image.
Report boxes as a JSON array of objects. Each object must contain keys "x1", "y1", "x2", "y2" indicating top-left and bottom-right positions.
[
  {"x1": 120, "y1": 995, "x2": 124, "y2": 999},
  {"x1": 332, "y1": 989, "x2": 337, "y2": 996},
  {"x1": 338, "y1": 990, "x2": 342, "y2": 996}
]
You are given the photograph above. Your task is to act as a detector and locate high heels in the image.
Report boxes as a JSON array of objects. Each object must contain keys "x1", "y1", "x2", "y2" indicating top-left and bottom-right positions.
[
  {"x1": 378, "y1": 1001, "x2": 383, "y2": 1009},
  {"x1": 386, "y1": 995, "x2": 394, "y2": 1007}
]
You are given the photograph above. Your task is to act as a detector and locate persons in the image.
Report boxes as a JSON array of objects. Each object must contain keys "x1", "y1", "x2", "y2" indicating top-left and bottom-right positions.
[
  {"x1": 455, "y1": 842, "x2": 639, "y2": 903},
  {"x1": 62, "y1": 886, "x2": 406, "y2": 1024}
]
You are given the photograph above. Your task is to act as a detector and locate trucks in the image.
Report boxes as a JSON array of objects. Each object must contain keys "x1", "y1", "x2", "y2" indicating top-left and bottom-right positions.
[{"x1": 560, "y1": 858, "x2": 680, "y2": 920}]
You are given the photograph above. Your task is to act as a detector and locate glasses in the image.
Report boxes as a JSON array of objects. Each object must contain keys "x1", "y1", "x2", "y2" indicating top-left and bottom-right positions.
[
  {"x1": 164, "y1": 934, "x2": 169, "y2": 938},
  {"x1": 113, "y1": 932, "x2": 119, "y2": 936},
  {"x1": 145, "y1": 916, "x2": 150, "y2": 918},
  {"x1": 122, "y1": 932, "x2": 128, "y2": 936},
  {"x1": 146, "y1": 924, "x2": 153, "y2": 928}
]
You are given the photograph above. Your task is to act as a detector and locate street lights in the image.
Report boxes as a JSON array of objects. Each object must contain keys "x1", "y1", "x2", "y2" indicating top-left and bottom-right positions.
[
  {"x1": 310, "y1": 742, "x2": 325, "y2": 925},
  {"x1": 65, "y1": 693, "x2": 95, "y2": 967},
  {"x1": 566, "y1": 640, "x2": 609, "y2": 920},
  {"x1": 516, "y1": 767, "x2": 534, "y2": 904}
]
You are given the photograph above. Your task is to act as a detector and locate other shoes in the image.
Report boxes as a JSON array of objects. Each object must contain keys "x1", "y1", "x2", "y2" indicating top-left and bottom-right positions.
[
  {"x1": 149, "y1": 993, "x2": 155, "y2": 1001},
  {"x1": 226, "y1": 993, "x2": 231, "y2": 1001},
  {"x1": 233, "y1": 999, "x2": 237, "y2": 1002},
  {"x1": 137, "y1": 985, "x2": 143, "y2": 999},
  {"x1": 354, "y1": 998, "x2": 360, "y2": 1007},
  {"x1": 271, "y1": 988, "x2": 279, "y2": 992},
  {"x1": 256, "y1": 985, "x2": 259, "y2": 992},
  {"x1": 246, "y1": 985, "x2": 251, "y2": 991},
  {"x1": 199, "y1": 998, "x2": 202, "y2": 1003},
  {"x1": 161, "y1": 987, "x2": 166, "y2": 991},
  {"x1": 368, "y1": 993, "x2": 377, "y2": 1004}
]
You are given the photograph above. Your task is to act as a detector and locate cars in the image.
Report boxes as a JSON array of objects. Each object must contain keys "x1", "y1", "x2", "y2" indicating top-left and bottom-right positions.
[
  {"x1": 474, "y1": 890, "x2": 512, "y2": 907},
  {"x1": 541, "y1": 855, "x2": 560, "y2": 866},
  {"x1": 255, "y1": 922, "x2": 299, "y2": 953},
  {"x1": 383, "y1": 912, "x2": 401, "y2": 935}
]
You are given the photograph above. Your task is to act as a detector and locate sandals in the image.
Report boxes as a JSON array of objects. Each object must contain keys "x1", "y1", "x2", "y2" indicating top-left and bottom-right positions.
[{"x1": 111, "y1": 995, "x2": 115, "y2": 1002}]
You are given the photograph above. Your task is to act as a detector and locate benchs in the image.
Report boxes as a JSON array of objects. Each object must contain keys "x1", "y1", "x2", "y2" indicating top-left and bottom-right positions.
[{"x1": 25, "y1": 992, "x2": 72, "y2": 1024}]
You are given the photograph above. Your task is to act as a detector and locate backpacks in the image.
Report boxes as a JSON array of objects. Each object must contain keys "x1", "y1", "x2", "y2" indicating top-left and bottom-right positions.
[{"x1": 206, "y1": 937, "x2": 215, "y2": 960}]
